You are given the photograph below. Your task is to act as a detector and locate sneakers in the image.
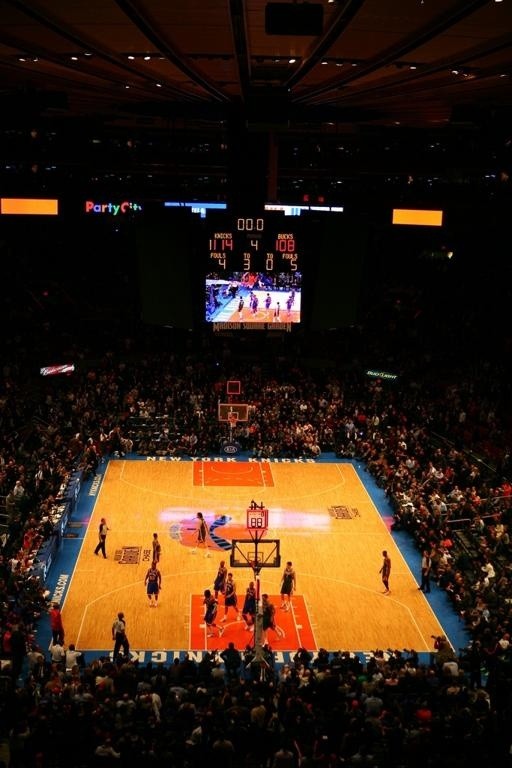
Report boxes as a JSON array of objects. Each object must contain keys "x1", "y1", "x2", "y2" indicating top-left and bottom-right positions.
[
  {"x1": 207, "y1": 633, "x2": 214, "y2": 637},
  {"x1": 219, "y1": 626, "x2": 225, "y2": 637},
  {"x1": 280, "y1": 601, "x2": 292, "y2": 611},
  {"x1": 236, "y1": 615, "x2": 241, "y2": 620},
  {"x1": 220, "y1": 615, "x2": 228, "y2": 622},
  {"x1": 149, "y1": 599, "x2": 159, "y2": 607}
]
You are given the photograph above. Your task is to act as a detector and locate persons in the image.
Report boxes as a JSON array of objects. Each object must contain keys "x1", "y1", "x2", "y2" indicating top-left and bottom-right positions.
[{"x1": 205, "y1": 269, "x2": 301, "y2": 322}]
